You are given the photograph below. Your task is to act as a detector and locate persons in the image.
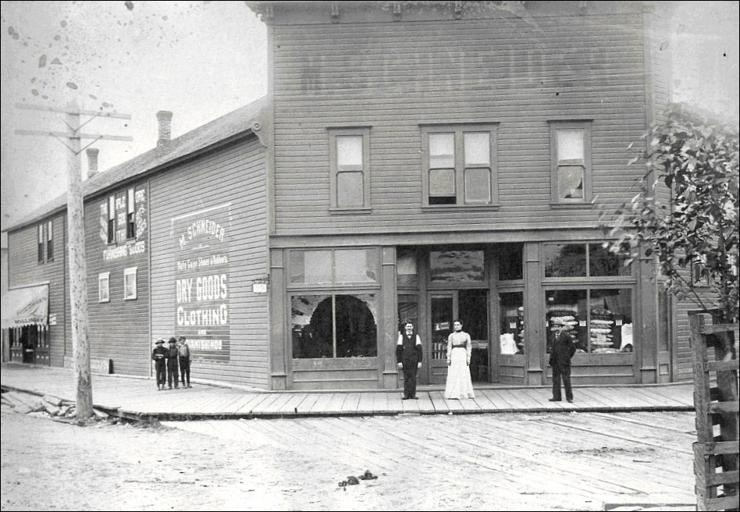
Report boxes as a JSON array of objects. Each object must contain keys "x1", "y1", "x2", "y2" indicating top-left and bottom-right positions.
[
  {"x1": 443, "y1": 319, "x2": 477, "y2": 400},
  {"x1": 396, "y1": 320, "x2": 424, "y2": 401},
  {"x1": 178, "y1": 336, "x2": 192, "y2": 389},
  {"x1": 168, "y1": 336, "x2": 179, "y2": 391},
  {"x1": 150, "y1": 338, "x2": 170, "y2": 389},
  {"x1": 547, "y1": 317, "x2": 575, "y2": 403}
]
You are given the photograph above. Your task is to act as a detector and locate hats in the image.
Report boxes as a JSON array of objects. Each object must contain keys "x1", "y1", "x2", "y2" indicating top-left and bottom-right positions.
[
  {"x1": 181, "y1": 336, "x2": 186, "y2": 340},
  {"x1": 168, "y1": 337, "x2": 177, "y2": 343},
  {"x1": 155, "y1": 339, "x2": 165, "y2": 344},
  {"x1": 551, "y1": 318, "x2": 565, "y2": 326}
]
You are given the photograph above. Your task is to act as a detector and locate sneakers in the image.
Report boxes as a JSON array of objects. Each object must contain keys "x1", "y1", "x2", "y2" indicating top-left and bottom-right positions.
[
  {"x1": 401, "y1": 395, "x2": 409, "y2": 400},
  {"x1": 567, "y1": 399, "x2": 574, "y2": 404},
  {"x1": 411, "y1": 395, "x2": 419, "y2": 399},
  {"x1": 157, "y1": 383, "x2": 193, "y2": 391},
  {"x1": 549, "y1": 397, "x2": 561, "y2": 401}
]
